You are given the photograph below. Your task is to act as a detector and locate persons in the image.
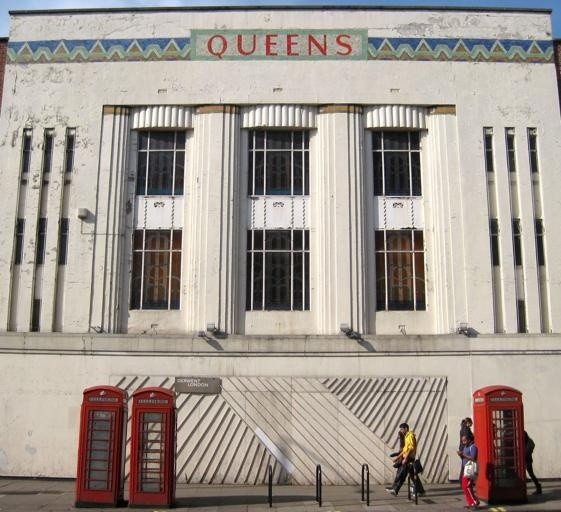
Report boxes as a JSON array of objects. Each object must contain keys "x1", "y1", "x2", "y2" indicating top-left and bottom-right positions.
[
  {"x1": 384, "y1": 428, "x2": 419, "y2": 493},
  {"x1": 523, "y1": 430, "x2": 542, "y2": 496},
  {"x1": 456, "y1": 432, "x2": 481, "y2": 511},
  {"x1": 458, "y1": 416, "x2": 474, "y2": 488},
  {"x1": 385, "y1": 422, "x2": 425, "y2": 498}
]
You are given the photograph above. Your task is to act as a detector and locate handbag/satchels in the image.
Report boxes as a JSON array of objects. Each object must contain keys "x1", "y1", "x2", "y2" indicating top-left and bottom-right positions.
[
  {"x1": 462, "y1": 460, "x2": 477, "y2": 479},
  {"x1": 413, "y1": 459, "x2": 425, "y2": 474}
]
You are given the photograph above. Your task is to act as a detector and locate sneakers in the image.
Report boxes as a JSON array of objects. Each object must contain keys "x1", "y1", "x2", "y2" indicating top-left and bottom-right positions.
[{"x1": 385, "y1": 486, "x2": 425, "y2": 497}]
